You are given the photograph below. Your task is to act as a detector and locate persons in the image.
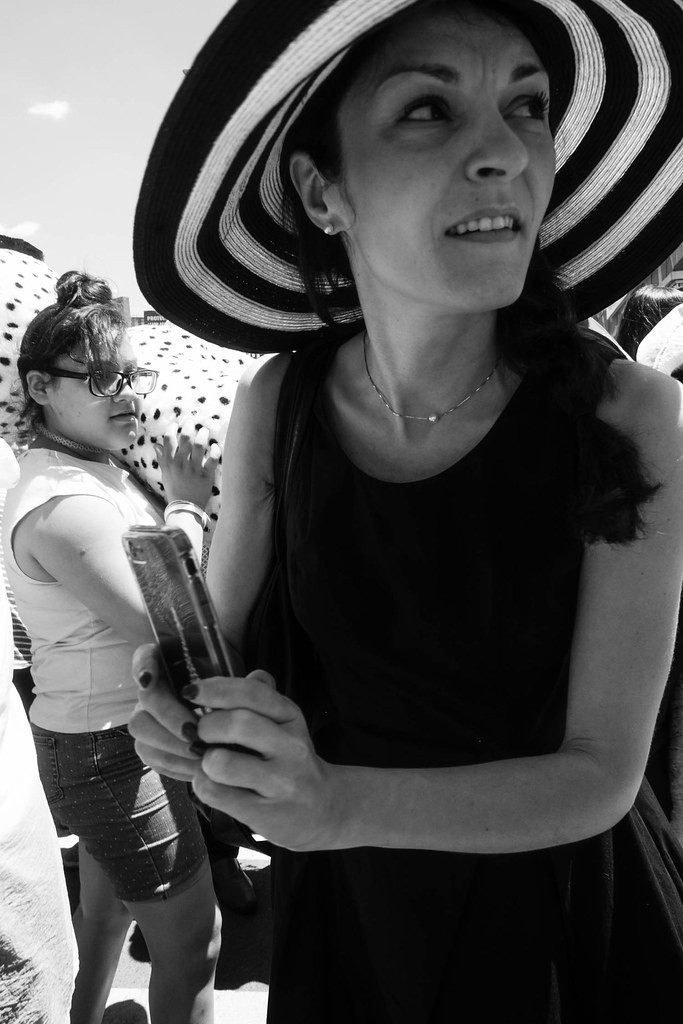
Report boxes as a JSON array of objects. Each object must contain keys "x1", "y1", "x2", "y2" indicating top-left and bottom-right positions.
[
  {"x1": 129, "y1": 1, "x2": 683, "y2": 1024},
  {"x1": 1, "y1": 230, "x2": 683, "y2": 1024}
]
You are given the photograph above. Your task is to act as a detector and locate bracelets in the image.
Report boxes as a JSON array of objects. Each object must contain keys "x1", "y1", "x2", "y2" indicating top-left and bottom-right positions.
[{"x1": 164, "y1": 499, "x2": 211, "y2": 528}]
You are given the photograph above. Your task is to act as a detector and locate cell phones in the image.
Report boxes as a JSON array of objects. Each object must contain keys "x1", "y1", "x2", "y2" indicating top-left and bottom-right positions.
[{"x1": 121, "y1": 525, "x2": 241, "y2": 721}]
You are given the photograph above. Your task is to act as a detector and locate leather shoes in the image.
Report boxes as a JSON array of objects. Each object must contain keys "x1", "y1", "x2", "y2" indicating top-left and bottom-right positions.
[{"x1": 209, "y1": 856, "x2": 259, "y2": 913}]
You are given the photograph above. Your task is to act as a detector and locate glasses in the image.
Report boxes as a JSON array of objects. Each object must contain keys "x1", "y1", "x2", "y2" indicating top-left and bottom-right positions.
[{"x1": 36, "y1": 366, "x2": 160, "y2": 398}]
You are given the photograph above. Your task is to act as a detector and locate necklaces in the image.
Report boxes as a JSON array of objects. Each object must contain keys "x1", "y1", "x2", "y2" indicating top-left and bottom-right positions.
[
  {"x1": 34, "y1": 422, "x2": 107, "y2": 454},
  {"x1": 362, "y1": 330, "x2": 502, "y2": 423}
]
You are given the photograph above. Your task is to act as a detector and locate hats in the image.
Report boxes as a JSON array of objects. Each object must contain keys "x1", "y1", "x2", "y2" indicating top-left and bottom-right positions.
[{"x1": 131, "y1": 0, "x2": 682, "y2": 352}]
